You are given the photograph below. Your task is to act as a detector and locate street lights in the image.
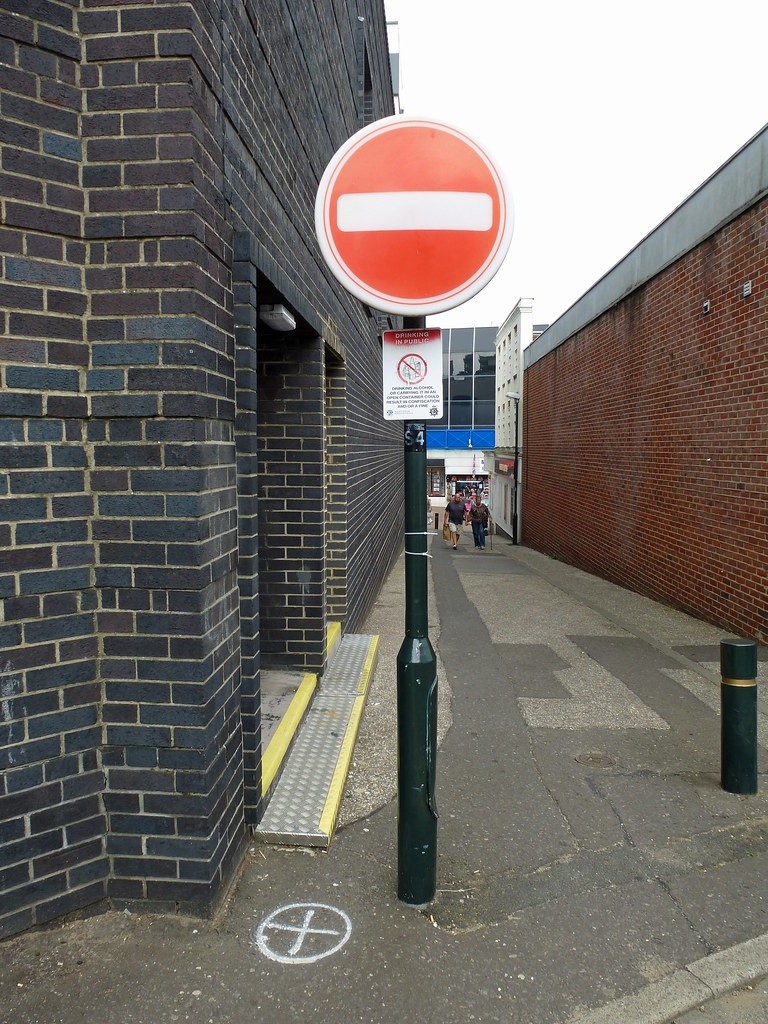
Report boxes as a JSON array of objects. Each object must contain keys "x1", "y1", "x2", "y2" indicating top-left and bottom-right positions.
[{"x1": 505, "y1": 391, "x2": 522, "y2": 547}]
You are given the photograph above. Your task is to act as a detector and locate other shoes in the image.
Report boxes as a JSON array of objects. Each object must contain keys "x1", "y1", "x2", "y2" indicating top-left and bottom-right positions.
[
  {"x1": 453, "y1": 544, "x2": 457, "y2": 550},
  {"x1": 476, "y1": 546, "x2": 480, "y2": 549},
  {"x1": 481, "y1": 546, "x2": 484, "y2": 550}
]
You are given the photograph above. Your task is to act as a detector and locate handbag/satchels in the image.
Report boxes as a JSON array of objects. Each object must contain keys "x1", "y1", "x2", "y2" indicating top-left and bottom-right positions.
[{"x1": 443, "y1": 524, "x2": 451, "y2": 542}]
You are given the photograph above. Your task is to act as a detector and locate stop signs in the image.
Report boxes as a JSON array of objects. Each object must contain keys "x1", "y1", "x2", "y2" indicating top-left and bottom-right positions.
[{"x1": 312, "y1": 110, "x2": 516, "y2": 317}]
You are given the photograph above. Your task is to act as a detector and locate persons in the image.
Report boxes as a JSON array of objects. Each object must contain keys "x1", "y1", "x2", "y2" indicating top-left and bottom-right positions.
[
  {"x1": 455, "y1": 484, "x2": 489, "y2": 525},
  {"x1": 468, "y1": 495, "x2": 492, "y2": 550},
  {"x1": 443, "y1": 494, "x2": 466, "y2": 549}
]
